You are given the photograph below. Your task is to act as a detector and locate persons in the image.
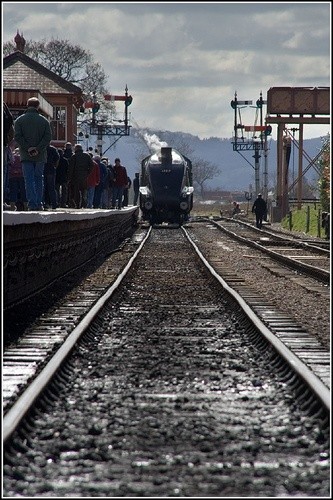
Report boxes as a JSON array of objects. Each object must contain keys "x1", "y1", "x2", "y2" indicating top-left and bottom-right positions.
[
  {"x1": 13, "y1": 97, "x2": 50, "y2": 212},
  {"x1": 6, "y1": 125, "x2": 132, "y2": 209},
  {"x1": 233, "y1": 202, "x2": 240, "y2": 216},
  {"x1": 133, "y1": 173, "x2": 140, "y2": 206},
  {"x1": 3, "y1": 101, "x2": 17, "y2": 210},
  {"x1": 252, "y1": 193, "x2": 266, "y2": 230}
]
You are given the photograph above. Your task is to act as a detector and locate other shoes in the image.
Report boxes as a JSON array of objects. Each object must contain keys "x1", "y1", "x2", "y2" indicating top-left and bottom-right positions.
[
  {"x1": 28, "y1": 207, "x2": 35, "y2": 210},
  {"x1": 60, "y1": 205, "x2": 67, "y2": 208},
  {"x1": 19, "y1": 203, "x2": 25, "y2": 210},
  {"x1": 38, "y1": 206, "x2": 44, "y2": 210},
  {"x1": 3, "y1": 202, "x2": 17, "y2": 211},
  {"x1": 52, "y1": 205, "x2": 56, "y2": 209}
]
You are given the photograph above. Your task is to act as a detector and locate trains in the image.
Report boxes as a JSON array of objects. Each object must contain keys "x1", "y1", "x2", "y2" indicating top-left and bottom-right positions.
[{"x1": 139, "y1": 145, "x2": 194, "y2": 227}]
